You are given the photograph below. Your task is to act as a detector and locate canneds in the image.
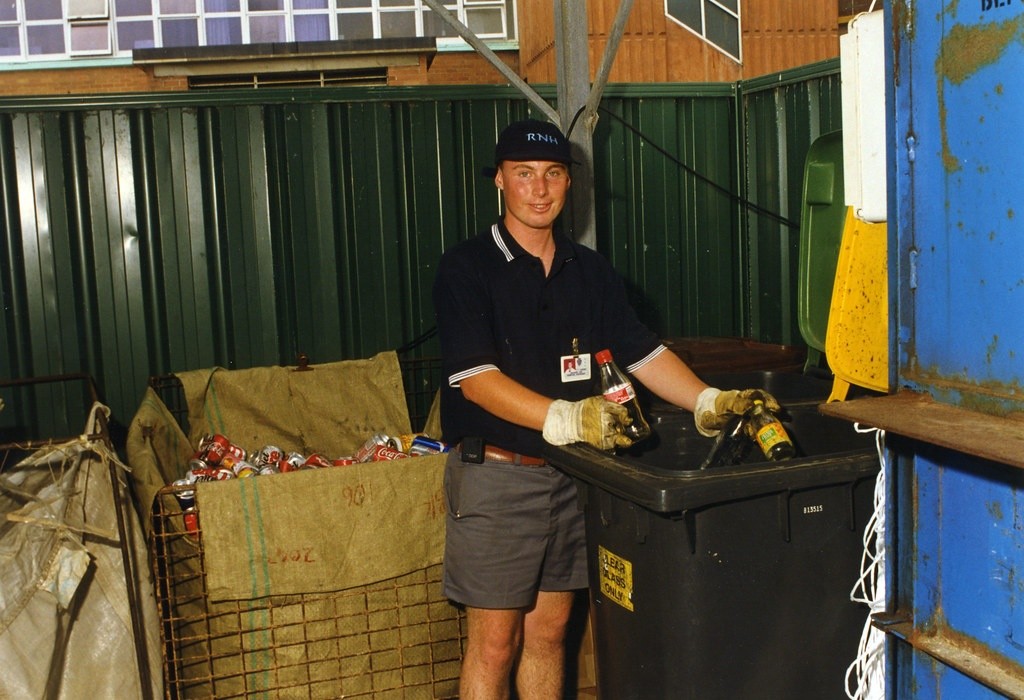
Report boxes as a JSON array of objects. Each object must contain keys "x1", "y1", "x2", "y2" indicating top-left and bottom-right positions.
[{"x1": 167, "y1": 431, "x2": 450, "y2": 538}]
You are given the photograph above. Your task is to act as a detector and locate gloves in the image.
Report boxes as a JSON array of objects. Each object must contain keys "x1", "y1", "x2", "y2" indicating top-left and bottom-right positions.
[
  {"x1": 543, "y1": 396, "x2": 633, "y2": 449},
  {"x1": 694, "y1": 386, "x2": 781, "y2": 438}
]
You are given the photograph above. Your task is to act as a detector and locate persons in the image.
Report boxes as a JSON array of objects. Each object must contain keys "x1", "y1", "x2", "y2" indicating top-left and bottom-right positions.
[{"x1": 432, "y1": 120, "x2": 783, "y2": 700}]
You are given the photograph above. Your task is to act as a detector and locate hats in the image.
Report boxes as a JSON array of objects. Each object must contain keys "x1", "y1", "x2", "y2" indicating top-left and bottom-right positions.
[{"x1": 495, "y1": 118, "x2": 571, "y2": 167}]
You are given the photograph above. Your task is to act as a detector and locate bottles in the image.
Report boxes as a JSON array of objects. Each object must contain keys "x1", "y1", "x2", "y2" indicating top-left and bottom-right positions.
[
  {"x1": 751, "y1": 399, "x2": 794, "y2": 462},
  {"x1": 595, "y1": 349, "x2": 651, "y2": 444}
]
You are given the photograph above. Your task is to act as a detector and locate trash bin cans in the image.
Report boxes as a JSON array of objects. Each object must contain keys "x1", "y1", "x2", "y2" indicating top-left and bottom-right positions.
[
  {"x1": 537, "y1": 204, "x2": 893, "y2": 700},
  {"x1": 139, "y1": 356, "x2": 470, "y2": 700},
  {"x1": 1, "y1": 371, "x2": 165, "y2": 700},
  {"x1": 656, "y1": 335, "x2": 828, "y2": 378},
  {"x1": 634, "y1": 128, "x2": 849, "y2": 415}
]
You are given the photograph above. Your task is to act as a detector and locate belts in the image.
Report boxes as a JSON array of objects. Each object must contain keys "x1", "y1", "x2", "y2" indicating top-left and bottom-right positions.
[{"x1": 450, "y1": 440, "x2": 546, "y2": 465}]
